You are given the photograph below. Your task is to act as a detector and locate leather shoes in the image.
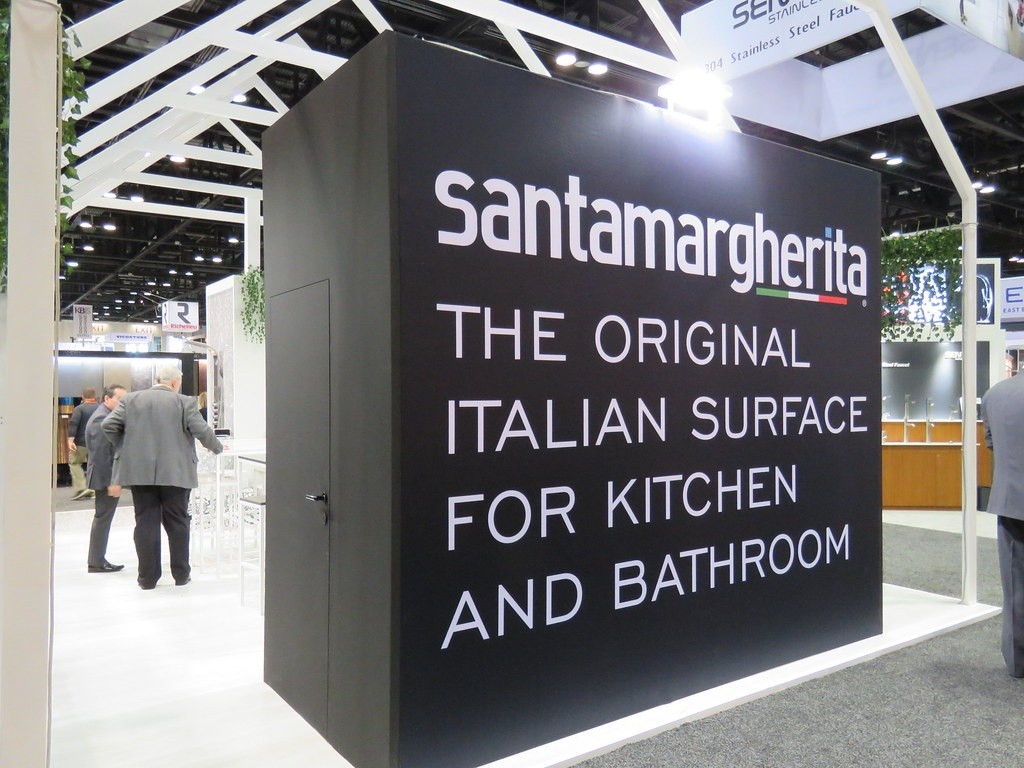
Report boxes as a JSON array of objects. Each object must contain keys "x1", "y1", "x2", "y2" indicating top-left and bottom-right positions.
[{"x1": 87, "y1": 560, "x2": 124, "y2": 572}]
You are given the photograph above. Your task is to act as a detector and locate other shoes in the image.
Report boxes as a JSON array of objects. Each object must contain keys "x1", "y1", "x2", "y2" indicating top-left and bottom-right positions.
[
  {"x1": 84, "y1": 490, "x2": 95, "y2": 497},
  {"x1": 72, "y1": 488, "x2": 90, "y2": 500}
]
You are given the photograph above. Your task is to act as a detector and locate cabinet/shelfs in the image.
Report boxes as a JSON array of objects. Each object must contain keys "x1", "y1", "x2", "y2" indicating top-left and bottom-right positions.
[{"x1": 188, "y1": 437, "x2": 266, "y2": 620}]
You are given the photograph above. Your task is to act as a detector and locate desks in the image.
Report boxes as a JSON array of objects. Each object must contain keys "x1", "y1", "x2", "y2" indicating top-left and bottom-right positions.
[{"x1": 880, "y1": 443, "x2": 981, "y2": 510}]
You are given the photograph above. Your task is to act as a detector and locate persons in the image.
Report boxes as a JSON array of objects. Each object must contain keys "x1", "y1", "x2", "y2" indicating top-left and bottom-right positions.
[
  {"x1": 981, "y1": 369, "x2": 1024, "y2": 678},
  {"x1": 101, "y1": 366, "x2": 224, "y2": 589},
  {"x1": 67, "y1": 388, "x2": 100, "y2": 500},
  {"x1": 198, "y1": 391, "x2": 207, "y2": 422},
  {"x1": 85, "y1": 384, "x2": 127, "y2": 573}
]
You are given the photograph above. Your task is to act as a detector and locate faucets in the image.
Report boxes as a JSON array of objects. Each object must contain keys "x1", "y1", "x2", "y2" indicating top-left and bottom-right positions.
[
  {"x1": 882, "y1": 394, "x2": 893, "y2": 421},
  {"x1": 925, "y1": 417, "x2": 934, "y2": 443},
  {"x1": 882, "y1": 429, "x2": 888, "y2": 445},
  {"x1": 903, "y1": 416, "x2": 916, "y2": 443},
  {"x1": 904, "y1": 394, "x2": 916, "y2": 418},
  {"x1": 925, "y1": 398, "x2": 935, "y2": 419},
  {"x1": 948, "y1": 404, "x2": 960, "y2": 420}
]
